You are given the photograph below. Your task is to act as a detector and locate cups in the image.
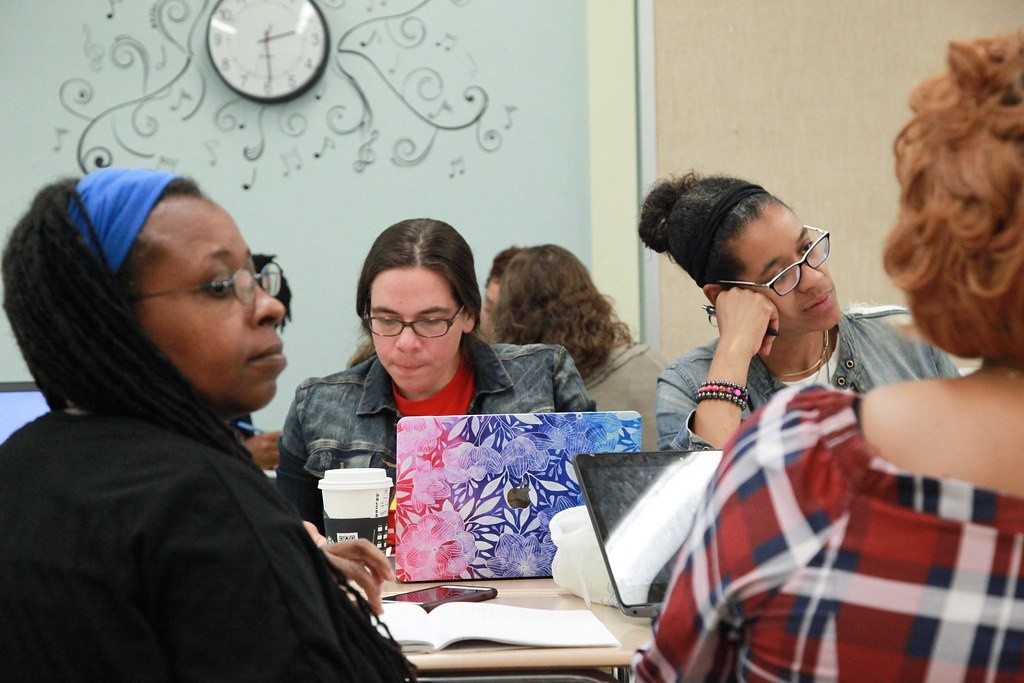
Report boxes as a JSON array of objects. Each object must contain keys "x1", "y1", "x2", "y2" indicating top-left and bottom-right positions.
[{"x1": 317, "y1": 467, "x2": 394, "y2": 558}]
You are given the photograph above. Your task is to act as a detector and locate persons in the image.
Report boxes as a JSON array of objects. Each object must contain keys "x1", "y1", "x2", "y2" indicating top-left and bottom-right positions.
[
  {"x1": 628, "y1": 26, "x2": 1024, "y2": 683},
  {"x1": 274, "y1": 218, "x2": 663, "y2": 615},
  {"x1": 0, "y1": 168, "x2": 417, "y2": 683},
  {"x1": 639, "y1": 169, "x2": 962, "y2": 451}
]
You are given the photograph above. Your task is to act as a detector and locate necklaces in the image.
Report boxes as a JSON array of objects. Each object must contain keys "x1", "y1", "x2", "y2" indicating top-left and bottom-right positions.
[
  {"x1": 774, "y1": 329, "x2": 830, "y2": 383},
  {"x1": 980, "y1": 365, "x2": 1024, "y2": 378}
]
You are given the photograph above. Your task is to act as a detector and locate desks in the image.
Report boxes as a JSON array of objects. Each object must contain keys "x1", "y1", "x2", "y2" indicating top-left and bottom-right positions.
[{"x1": 352, "y1": 577, "x2": 656, "y2": 683}]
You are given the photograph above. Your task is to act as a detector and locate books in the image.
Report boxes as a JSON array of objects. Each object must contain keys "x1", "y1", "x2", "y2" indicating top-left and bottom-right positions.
[{"x1": 371, "y1": 602, "x2": 623, "y2": 654}]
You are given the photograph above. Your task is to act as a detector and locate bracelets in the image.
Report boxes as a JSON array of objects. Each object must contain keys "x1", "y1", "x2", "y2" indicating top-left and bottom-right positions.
[{"x1": 695, "y1": 381, "x2": 750, "y2": 411}]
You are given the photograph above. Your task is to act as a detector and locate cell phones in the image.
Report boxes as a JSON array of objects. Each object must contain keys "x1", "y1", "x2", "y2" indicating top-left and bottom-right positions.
[{"x1": 382, "y1": 585, "x2": 498, "y2": 613}]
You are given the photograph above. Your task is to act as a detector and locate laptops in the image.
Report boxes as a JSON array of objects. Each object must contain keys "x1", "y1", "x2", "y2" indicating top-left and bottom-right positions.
[
  {"x1": 0, "y1": 382, "x2": 51, "y2": 448},
  {"x1": 574, "y1": 447, "x2": 723, "y2": 619},
  {"x1": 394, "y1": 410, "x2": 642, "y2": 582}
]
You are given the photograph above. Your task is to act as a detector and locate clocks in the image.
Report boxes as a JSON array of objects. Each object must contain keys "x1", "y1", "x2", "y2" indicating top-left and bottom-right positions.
[{"x1": 204, "y1": 1, "x2": 331, "y2": 105}]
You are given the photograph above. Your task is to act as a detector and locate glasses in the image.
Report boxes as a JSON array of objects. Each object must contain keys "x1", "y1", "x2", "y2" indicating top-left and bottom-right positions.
[
  {"x1": 135, "y1": 261, "x2": 284, "y2": 304},
  {"x1": 710, "y1": 225, "x2": 832, "y2": 297},
  {"x1": 364, "y1": 301, "x2": 467, "y2": 338}
]
